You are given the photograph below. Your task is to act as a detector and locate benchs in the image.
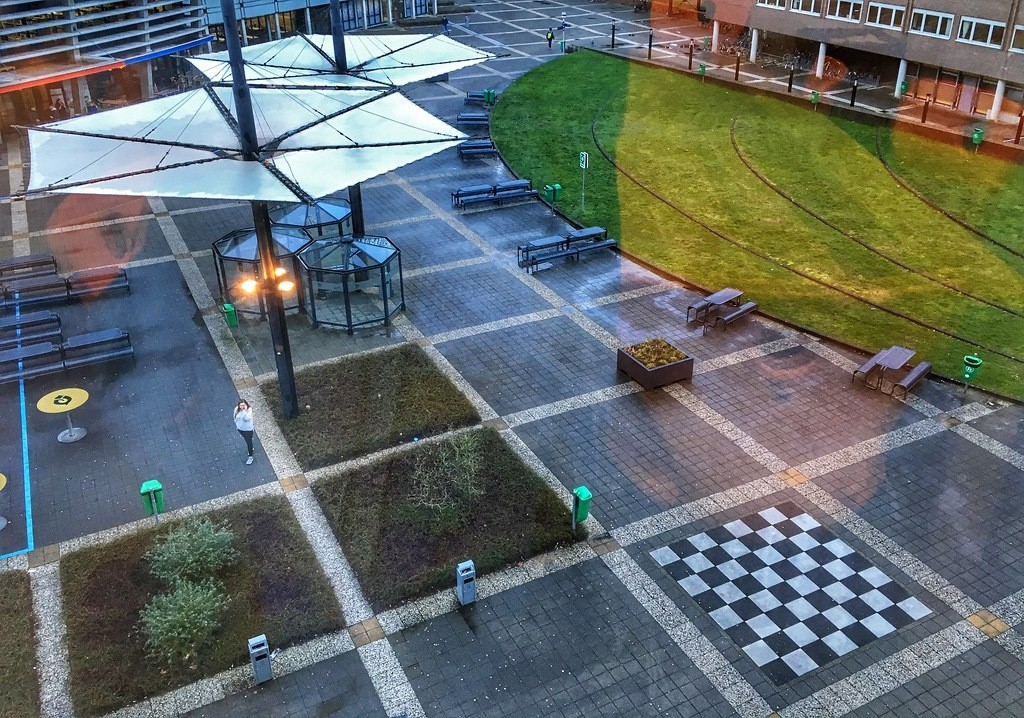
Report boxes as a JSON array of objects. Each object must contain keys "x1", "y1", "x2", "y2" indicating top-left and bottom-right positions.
[
  {"x1": 452, "y1": 183, "x2": 539, "y2": 211},
  {"x1": 518, "y1": 229, "x2": 617, "y2": 274},
  {"x1": 456, "y1": 90, "x2": 498, "y2": 159},
  {"x1": 686, "y1": 300, "x2": 758, "y2": 332},
  {"x1": 852, "y1": 348, "x2": 933, "y2": 400},
  {"x1": 0, "y1": 253, "x2": 136, "y2": 383}
]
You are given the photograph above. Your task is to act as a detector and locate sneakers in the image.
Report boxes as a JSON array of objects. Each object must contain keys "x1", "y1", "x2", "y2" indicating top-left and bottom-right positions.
[
  {"x1": 247, "y1": 447, "x2": 255, "y2": 455},
  {"x1": 246, "y1": 456, "x2": 254, "y2": 465}
]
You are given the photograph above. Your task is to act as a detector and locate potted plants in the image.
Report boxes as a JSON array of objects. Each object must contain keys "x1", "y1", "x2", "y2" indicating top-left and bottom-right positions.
[{"x1": 617, "y1": 338, "x2": 695, "y2": 392}]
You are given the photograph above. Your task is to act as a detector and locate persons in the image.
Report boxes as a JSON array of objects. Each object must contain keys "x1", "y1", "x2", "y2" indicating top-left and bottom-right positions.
[
  {"x1": 233, "y1": 399, "x2": 253, "y2": 465},
  {"x1": 28, "y1": 96, "x2": 98, "y2": 123},
  {"x1": 546, "y1": 27, "x2": 554, "y2": 48},
  {"x1": 442, "y1": 16, "x2": 450, "y2": 35}
]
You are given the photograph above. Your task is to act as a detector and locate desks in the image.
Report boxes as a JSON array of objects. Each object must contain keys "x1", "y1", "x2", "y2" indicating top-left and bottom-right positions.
[
  {"x1": 457, "y1": 184, "x2": 492, "y2": 204},
  {"x1": 0, "y1": 341, "x2": 58, "y2": 363},
  {"x1": 467, "y1": 91, "x2": 484, "y2": 98},
  {"x1": 67, "y1": 266, "x2": 126, "y2": 290},
  {"x1": 37, "y1": 388, "x2": 89, "y2": 443},
  {"x1": 460, "y1": 141, "x2": 494, "y2": 150},
  {"x1": 0, "y1": 310, "x2": 60, "y2": 332},
  {"x1": 0, "y1": 254, "x2": 53, "y2": 272},
  {"x1": 875, "y1": 346, "x2": 917, "y2": 391},
  {"x1": 493, "y1": 178, "x2": 533, "y2": 195},
  {"x1": 526, "y1": 236, "x2": 566, "y2": 262},
  {"x1": 62, "y1": 327, "x2": 129, "y2": 355},
  {"x1": 567, "y1": 226, "x2": 608, "y2": 249},
  {"x1": 703, "y1": 287, "x2": 744, "y2": 323},
  {"x1": 457, "y1": 113, "x2": 489, "y2": 121},
  {"x1": 3, "y1": 273, "x2": 68, "y2": 297}
]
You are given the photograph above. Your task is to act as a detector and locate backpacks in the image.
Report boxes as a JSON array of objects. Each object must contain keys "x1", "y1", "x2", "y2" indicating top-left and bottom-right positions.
[{"x1": 548, "y1": 32, "x2": 552, "y2": 39}]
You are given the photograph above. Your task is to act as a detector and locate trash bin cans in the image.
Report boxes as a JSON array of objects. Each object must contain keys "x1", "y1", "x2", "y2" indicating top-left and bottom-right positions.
[
  {"x1": 901, "y1": 81, "x2": 909, "y2": 93},
  {"x1": 704, "y1": 35, "x2": 709, "y2": 45},
  {"x1": 963, "y1": 354, "x2": 983, "y2": 382},
  {"x1": 483, "y1": 89, "x2": 495, "y2": 103},
  {"x1": 247, "y1": 633, "x2": 274, "y2": 685},
  {"x1": 544, "y1": 183, "x2": 562, "y2": 202},
  {"x1": 699, "y1": 63, "x2": 705, "y2": 75},
  {"x1": 457, "y1": 559, "x2": 476, "y2": 606},
  {"x1": 140, "y1": 479, "x2": 165, "y2": 515},
  {"x1": 811, "y1": 90, "x2": 819, "y2": 103},
  {"x1": 222, "y1": 303, "x2": 237, "y2": 327},
  {"x1": 560, "y1": 41, "x2": 566, "y2": 50},
  {"x1": 973, "y1": 127, "x2": 983, "y2": 144},
  {"x1": 573, "y1": 485, "x2": 593, "y2": 522}
]
